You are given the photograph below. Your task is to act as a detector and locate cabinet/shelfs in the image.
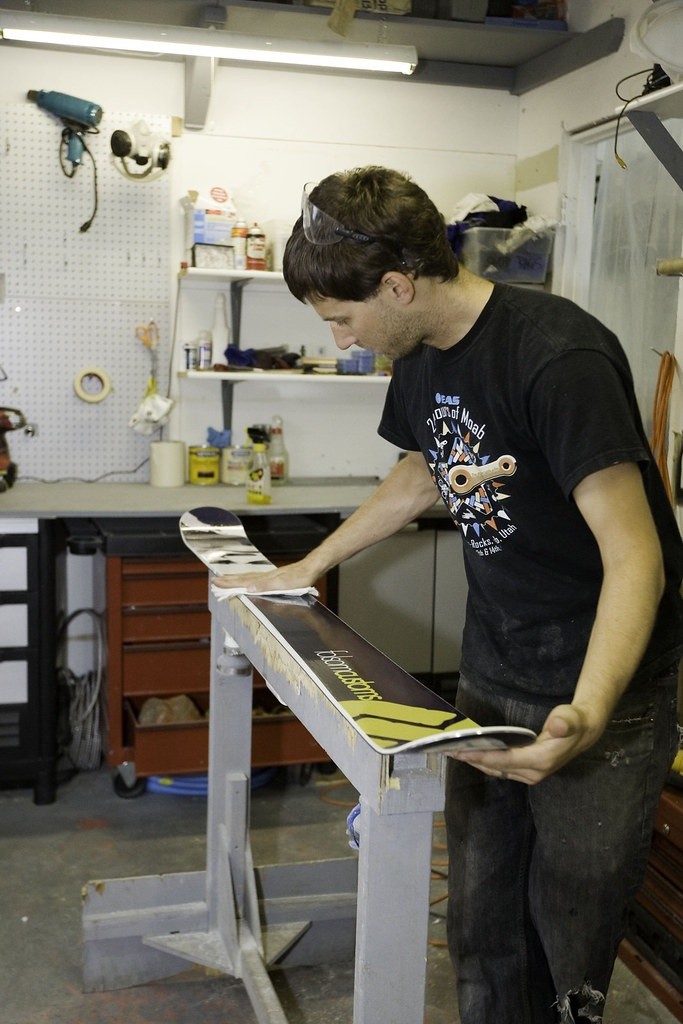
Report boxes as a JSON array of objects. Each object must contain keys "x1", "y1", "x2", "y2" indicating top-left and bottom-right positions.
[{"x1": 177, "y1": 268, "x2": 392, "y2": 445}]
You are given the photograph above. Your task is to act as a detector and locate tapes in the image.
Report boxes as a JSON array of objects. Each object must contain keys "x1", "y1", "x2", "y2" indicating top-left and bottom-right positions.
[{"x1": 75, "y1": 367, "x2": 110, "y2": 402}]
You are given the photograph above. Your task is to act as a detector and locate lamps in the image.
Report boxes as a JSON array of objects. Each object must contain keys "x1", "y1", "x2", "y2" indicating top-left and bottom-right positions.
[{"x1": 0, "y1": 8, "x2": 418, "y2": 76}]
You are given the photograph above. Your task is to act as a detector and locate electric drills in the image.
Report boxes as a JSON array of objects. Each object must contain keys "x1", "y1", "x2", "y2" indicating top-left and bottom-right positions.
[{"x1": 27, "y1": 88, "x2": 101, "y2": 175}]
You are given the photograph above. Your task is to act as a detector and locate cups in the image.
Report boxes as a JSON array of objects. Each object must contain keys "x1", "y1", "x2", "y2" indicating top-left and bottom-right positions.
[{"x1": 150, "y1": 441, "x2": 185, "y2": 487}]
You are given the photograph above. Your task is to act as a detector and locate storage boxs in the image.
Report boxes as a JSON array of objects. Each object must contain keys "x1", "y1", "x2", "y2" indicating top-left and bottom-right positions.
[{"x1": 462, "y1": 227, "x2": 552, "y2": 283}]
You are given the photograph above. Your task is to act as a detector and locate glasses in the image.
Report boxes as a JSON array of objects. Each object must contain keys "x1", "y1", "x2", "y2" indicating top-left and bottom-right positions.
[{"x1": 302, "y1": 182, "x2": 406, "y2": 265}]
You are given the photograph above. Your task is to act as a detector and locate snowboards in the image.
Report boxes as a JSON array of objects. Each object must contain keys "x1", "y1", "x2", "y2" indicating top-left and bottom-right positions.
[{"x1": 178, "y1": 505, "x2": 537, "y2": 756}]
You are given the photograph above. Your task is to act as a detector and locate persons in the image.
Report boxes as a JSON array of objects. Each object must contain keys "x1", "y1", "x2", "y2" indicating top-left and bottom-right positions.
[{"x1": 213, "y1": 166, "x2": 683, "y2": 1024}]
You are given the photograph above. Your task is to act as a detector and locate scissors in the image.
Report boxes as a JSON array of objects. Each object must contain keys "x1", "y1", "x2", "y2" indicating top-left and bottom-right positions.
[{"x1": 135, "y1": 320, "x2": 158, "y2": 385}]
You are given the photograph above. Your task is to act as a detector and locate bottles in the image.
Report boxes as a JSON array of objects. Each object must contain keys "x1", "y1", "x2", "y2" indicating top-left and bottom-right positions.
[
  {"x1": 244, "y1": 223, "x2": 266, "y2": 269},
  {"x1": 247, "y1": 444, "x2": 273, "y2": 504},
  {"x1": 269, "y1": 417, "x2": 289, "y2": 485},
  {"x1": 231, "y1": 215, "x2": 248, "y2": 270}
]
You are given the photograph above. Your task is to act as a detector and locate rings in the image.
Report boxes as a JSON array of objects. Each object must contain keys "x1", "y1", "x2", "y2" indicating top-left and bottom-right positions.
[{"x1": 500, "y1": 771, "x2": 508, "y2": 783}]
